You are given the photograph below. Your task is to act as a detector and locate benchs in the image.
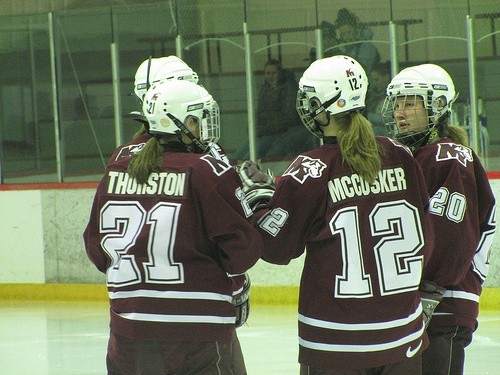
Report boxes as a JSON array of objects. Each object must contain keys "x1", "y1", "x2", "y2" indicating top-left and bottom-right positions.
[{"x1": 0, "y1": 0, "x2": 500, "y2": 183}]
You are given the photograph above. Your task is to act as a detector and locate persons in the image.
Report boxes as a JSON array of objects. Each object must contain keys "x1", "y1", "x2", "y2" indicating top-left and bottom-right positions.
[
  {"x1": 84, "y1": 55, "x2": 252, "y2": 327},
  {"x1": 237, "y1": 8, "x2": 387, "y2": 166},
  {"x1": 236, "y1": 54, "x2": 433, "y2": 375},
  {"x1": 381, "y1": 63, "x2": 497, "y2": 375},
  {"x1": 82, "y1": 79, "x2": 264, "y2": 375}
]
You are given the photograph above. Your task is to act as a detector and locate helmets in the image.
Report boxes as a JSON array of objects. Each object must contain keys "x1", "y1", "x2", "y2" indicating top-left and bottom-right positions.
[
  {"x1": 381, "y1": 64, "x2": 455, "y2": 154},
  {"x1": 295, "y1": 55, "x2": 369, "y2": 139},
  {"x1": 134, "y1": 56, "x2": 199, "y2": 103},
  {"x1": 142, "y1": 79, "x2": 220, "y2": 154}
]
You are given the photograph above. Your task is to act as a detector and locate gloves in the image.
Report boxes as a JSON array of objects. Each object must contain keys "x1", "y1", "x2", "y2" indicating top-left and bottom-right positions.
[
  {"x1": 419, "y1": 280, "x2": 447, "y2": 329},
  {"x1": 235, "y1": 161, "x2": 276, "y2": 204},
  {"x1": 232, "y1": 273, "x2": 251, "y2": 328}
]
